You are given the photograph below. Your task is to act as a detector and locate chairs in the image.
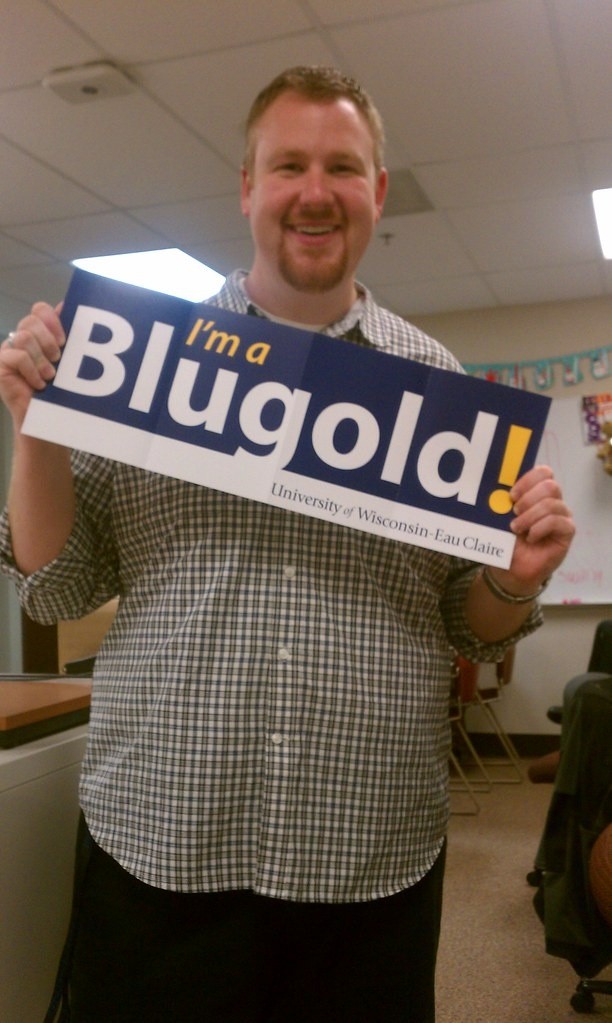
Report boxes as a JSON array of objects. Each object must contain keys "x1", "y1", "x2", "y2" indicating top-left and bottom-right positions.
[
  {"x1": 450, "y1": 643, "x2": 526, "y2": 784},
  {"x1": 448, "y1": 653, "x2": 495, "y2": 816}
]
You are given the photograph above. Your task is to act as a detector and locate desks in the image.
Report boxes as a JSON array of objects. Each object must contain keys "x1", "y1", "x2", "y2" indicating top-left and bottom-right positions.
[{"x1": 0, "y1": 725, "x2": 88, "y2": 1023}]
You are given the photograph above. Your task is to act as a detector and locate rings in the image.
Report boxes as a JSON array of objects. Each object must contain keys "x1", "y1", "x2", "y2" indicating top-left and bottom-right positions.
[{"x1": 8, "y1": 332, "x2": 14, "y2": 347}]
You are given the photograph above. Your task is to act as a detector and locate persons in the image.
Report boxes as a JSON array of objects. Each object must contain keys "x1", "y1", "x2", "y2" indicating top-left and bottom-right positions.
[
  {"x1": 0, "y1": 64, "x2": 576, "y2": 1023},
  {"x1": 527, "y1": 735, "x2": 612, "y2": 926}
]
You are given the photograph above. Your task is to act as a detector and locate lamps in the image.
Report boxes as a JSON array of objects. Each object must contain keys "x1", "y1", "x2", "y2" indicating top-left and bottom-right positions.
[{"x1": 42, "y1": 60, "x2": 136, "y2": 105}]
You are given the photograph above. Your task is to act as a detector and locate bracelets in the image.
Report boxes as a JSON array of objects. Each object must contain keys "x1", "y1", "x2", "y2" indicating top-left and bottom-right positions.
[{"x1": 481, "y1": 565, "x2": 552, "y2": 603}]
